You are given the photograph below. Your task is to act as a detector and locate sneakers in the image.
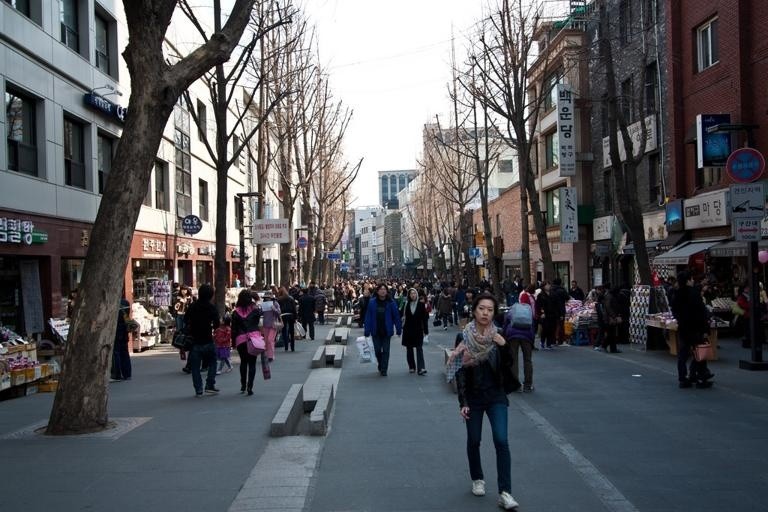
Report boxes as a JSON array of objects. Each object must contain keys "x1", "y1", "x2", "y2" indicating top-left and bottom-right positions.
[
  {"x1": 377, "y1": 364, "x2": 388, "y2": 377},
  {"x1": 417, "y1": 369, "x2": 428, "y2": 375},
  {"x1": 196, "y1": 385, "x2": 220, "y2": 398},
  {"x1": 108, "y1": 377, "x2": 131, "y2": 383},
  {"x1": 517, "y1": 385, "x2": 535, "y2": 393},
  {"x1": 444, "y1": 326, "x2": 447, "y2": 331},
  {"x1": 240, "y1": 386, "x2": 253, "y2": 396},
  {"x1": 409, "y1": 368, "x2": 414, "y2": 373},
  {"x1": 678, "y1": 372, "x2": 716, "y2": 390},
  {"x1": 472, "y1": 479, "x2": 487, "y2": 496},
  {"x1": 496, "y1": 491, "x2": 519, "y2": 509},
  {"x1": 531, "y1": 341, "x2": 569, "y2": 351}
]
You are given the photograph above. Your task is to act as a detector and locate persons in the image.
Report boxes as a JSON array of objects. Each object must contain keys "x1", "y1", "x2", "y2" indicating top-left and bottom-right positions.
[
  {"x1": 67, "y1": 290, "x2": 77, "y2": 317},
  {"x1": 107, "y1": 288, "x2": 132, "y2": 382},
  {"x1": 173, "y1": 271, "x2": 768, "y2": 395},
  {"x1": 453, "y1": 293, "x2": 520, "y2": 510}
]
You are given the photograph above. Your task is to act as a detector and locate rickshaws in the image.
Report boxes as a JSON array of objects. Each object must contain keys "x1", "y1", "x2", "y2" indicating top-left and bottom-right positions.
[{"x1": 351, "y1": 302, "x2": 367, "y2": 329}]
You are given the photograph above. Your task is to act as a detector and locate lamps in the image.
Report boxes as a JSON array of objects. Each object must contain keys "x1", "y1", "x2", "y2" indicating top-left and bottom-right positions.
[
  {"x1": 102, "y1": 89, "x2": 123, "y2": 97},
  {"x1": 90, "y1": 82, "x2": 115, "y2": 93}
]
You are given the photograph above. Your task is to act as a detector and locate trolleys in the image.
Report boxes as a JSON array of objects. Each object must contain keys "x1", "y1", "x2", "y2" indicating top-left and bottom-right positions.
[{"x1": 497, "y1": 302, "x2": 511, "y2": 329}]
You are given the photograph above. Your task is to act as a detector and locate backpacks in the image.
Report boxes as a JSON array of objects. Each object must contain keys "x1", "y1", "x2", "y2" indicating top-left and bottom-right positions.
[{"x1": 510, "y1": 302, "x2": 533, "y2": 330}]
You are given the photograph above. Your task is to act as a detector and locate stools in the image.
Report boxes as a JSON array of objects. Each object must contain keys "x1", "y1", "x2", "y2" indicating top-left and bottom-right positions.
[{"x1": 569, "y1": 326, "x2": 591, "y2": 347}]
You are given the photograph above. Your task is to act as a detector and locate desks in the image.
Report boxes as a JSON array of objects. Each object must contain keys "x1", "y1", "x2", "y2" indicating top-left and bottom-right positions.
[{"x1": 645, "y1": 317, "x2": 730, "y2": 332}]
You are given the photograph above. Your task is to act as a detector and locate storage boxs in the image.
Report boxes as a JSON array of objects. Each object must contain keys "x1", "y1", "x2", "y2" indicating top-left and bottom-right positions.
[
  {"x1": 666, "y1": 327, "x2": 718, "y2": 357},
  {"x1": 0, "y1": 341, "x2": 58, "y2": 394}
]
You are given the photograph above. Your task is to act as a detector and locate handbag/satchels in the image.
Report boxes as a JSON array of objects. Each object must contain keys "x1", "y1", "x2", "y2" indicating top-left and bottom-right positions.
[
  {"x1": 693, "y1": 343, "x2": 714, "y2": 361},
  {"x1": 246, "y1": 334, "x2": 267, "y2": 355}
]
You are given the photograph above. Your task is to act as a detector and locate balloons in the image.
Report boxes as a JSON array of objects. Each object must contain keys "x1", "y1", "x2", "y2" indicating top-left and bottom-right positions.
[{"x1": 758, "y1": 250, "x2": 768, "y2": 265}]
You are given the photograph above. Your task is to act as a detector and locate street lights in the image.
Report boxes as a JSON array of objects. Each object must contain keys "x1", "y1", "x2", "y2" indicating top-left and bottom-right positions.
[
  {"x1": 294, "y1": 226, "x2": 310, "y2": 286},
  {"x1": 440, "y1": 242, "x2": 454, "y2": 287}
]
